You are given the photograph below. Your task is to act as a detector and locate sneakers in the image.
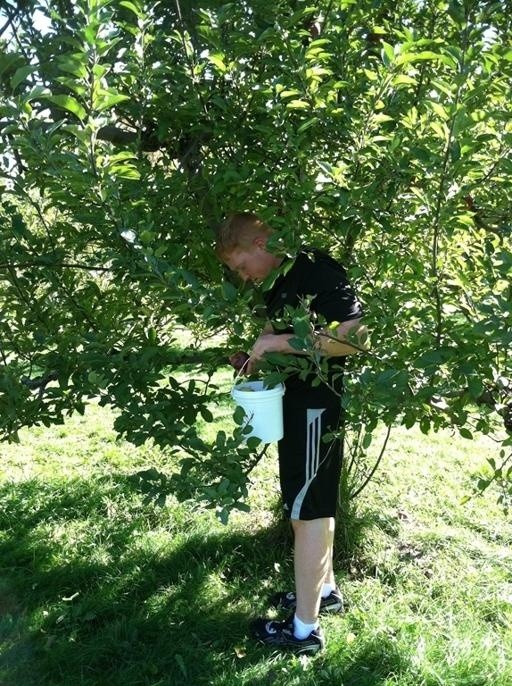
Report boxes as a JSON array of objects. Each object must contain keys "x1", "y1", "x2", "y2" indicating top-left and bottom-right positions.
[
  {"x1": 267, "y1": 585, "x2": 343, "y2": 617},
  {"x1": 248, "y1": 612, "x2": 325, "y2": 658}
]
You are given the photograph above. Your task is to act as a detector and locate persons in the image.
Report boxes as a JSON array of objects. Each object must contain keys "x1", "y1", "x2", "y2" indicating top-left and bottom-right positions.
[{"x1": 218, "y1": 212, "x2": 373, "y2": 657}]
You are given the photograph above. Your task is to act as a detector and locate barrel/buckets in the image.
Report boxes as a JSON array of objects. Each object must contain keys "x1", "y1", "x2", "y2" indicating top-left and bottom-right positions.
[{"x1": 232, "y1": 381, "x2": 287, "y2": 443}]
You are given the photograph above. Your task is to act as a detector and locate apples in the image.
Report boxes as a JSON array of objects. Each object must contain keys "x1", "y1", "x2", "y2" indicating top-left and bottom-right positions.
[
  {"x1": 229, "y1": 351, "x2": 250, "y2": 370},
  {"x1": 240, "y1": 386, "x2": 254, "y2": 392}
]
